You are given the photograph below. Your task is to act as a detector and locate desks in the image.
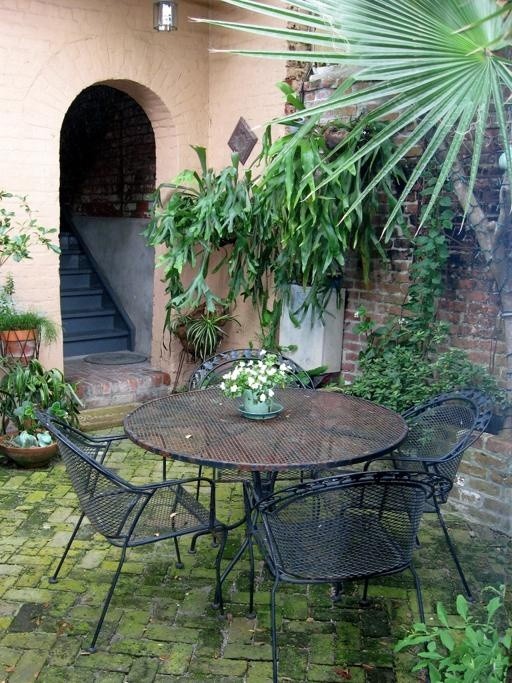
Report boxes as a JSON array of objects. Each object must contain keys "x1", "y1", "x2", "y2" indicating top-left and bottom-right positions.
[{"x1": 123, "y1": 383, "x2": 409, "y2": 624}]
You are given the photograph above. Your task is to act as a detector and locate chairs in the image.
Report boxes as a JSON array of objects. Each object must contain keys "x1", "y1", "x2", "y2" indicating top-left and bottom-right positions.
[
  {"x1": 35, "y1": 406, "x2": 230, "y2": 649},
  {"x1": 299, "y1": 384, "x2": 498, "y2": 604},
  {"x1": 215, "y1": 468, "x2": 456, "y2": 681},
  {"x1": 183, "y1": 347, "x2": 337, "y2": 538}
]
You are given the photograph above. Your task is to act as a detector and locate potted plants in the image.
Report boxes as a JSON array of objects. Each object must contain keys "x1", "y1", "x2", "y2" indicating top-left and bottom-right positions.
[
  {"x1": 2, "y1": 360, "x2": 79, "y2": 464},
  {"x1": 158, "y1": 305, "x2": 247, "y2": 356},
  {"x1": 0, "y1": 274, "x2": 60, "y2": 364}
]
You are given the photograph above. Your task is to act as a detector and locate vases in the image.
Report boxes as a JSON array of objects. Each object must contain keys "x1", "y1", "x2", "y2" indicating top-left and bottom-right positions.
[{"x1": 242, "y1": 385, "x2": 272, "y2": 416}]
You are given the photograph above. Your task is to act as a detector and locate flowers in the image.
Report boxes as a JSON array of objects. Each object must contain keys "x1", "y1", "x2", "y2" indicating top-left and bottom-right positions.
[{"x1": 215, "y1": 350, "x2": 310, "y2": 403}]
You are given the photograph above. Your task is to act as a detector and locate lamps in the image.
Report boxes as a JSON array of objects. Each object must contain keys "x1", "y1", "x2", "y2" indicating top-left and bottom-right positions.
[{"x1": 149, "y1": 0, "x2": 178, "y2": 35}]
